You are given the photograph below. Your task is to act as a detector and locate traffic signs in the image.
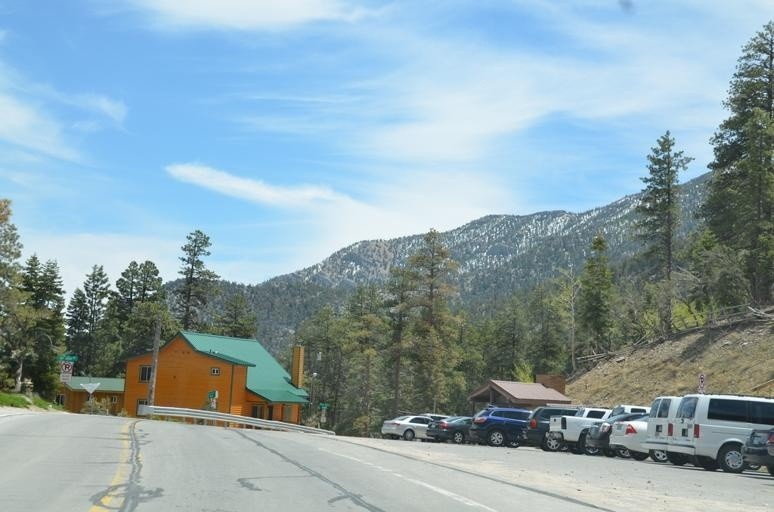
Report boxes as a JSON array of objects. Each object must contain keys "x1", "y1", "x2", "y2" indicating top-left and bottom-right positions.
[
  {"x1": 208, "y1": 390, "x2": 216, "y2": 399},
  {"x1": 319, "y1": 402, "x2": 331, "y2": 410},
  {"x1": 57, "y1": 354, "x2": 78, "y2": 361}
]
